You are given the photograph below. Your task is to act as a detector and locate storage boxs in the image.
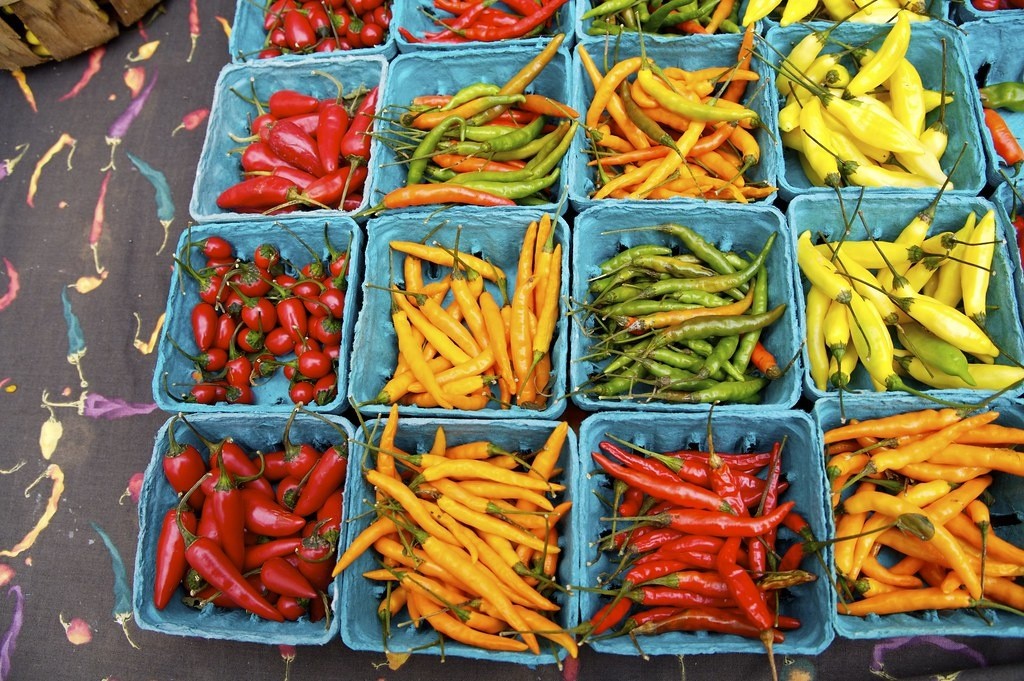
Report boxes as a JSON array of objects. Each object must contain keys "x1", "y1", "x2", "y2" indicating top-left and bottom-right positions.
[{"x1": 132, "y1": 0, "x2": 1024, "y2": 668}]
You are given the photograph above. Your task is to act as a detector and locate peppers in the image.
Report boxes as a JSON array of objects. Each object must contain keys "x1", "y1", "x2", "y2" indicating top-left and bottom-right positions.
[
  {"x1": 217, "y1": 1, "x2": 1023, "y2": 216},
  {"x1": 168, "y1": 209, "x2": 1024, "y2": 405},
  {"x1": 155, "y1": 406, "x2": 1024, "y2": 681}
]
